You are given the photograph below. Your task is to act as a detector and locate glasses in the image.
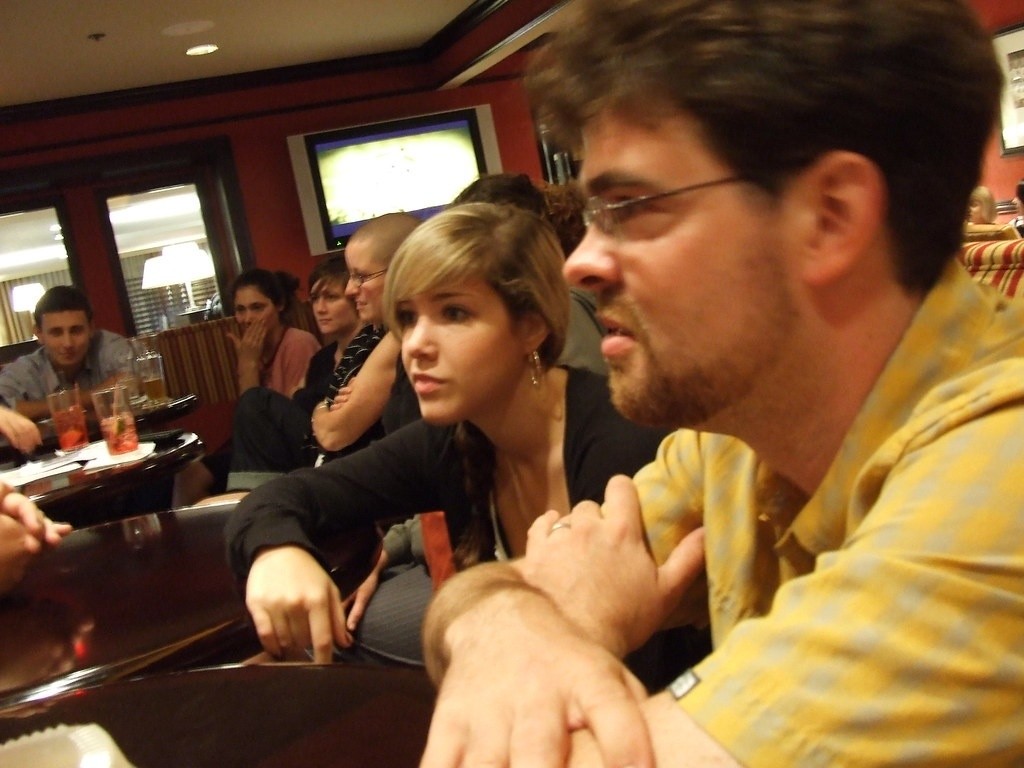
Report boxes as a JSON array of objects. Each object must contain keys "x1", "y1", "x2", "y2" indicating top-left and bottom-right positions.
[
  {"x1": 345, "y1": 268, "x2": 389, "y2": 287},
  {"x1": 582, "y1": 166, "x2": 812, "y2": 238}
]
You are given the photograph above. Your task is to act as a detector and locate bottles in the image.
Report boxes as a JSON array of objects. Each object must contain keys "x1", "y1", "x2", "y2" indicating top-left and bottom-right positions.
[
  {"x1": 537, "y1": 124, "x2": 558, "y2": 184},
  {"x1": 553, "y1": 153, "x2": 572, "y2": 185}
]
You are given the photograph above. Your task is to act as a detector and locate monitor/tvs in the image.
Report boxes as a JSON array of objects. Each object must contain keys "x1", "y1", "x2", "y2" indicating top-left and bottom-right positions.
[{"x1": 285, "y1": 104, "x2": 504, "y2": 257}]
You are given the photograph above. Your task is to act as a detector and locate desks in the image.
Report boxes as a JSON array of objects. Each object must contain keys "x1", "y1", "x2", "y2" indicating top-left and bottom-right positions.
[
  {"x1": 0, "y1": 502, "x2": 384, "y2": 706},
  {"x1": 0, "y1": 394, "x2": 200, "y2": 465},
  {"x1": 0, "y1": 432, "x2": 205, "y2": 532},
  {"x1": 0, "y1": 664, "x2": 437, "y2": 768}
]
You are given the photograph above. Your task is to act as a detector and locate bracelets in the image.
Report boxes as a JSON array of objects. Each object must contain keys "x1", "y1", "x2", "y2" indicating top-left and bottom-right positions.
[{"x1": 312, "y1": 405, "x2": 327, "y2": 422}]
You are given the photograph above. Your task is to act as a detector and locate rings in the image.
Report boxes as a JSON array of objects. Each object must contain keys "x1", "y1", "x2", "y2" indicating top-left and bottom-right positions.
[{"x1": 549, "y1": 523, "x2": 570, "y2": 534}]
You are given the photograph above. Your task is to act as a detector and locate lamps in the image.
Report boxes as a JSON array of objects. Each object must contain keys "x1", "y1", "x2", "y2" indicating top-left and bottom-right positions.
[
  {"x1": 141, "y1": 249, "x2": 215, "y2": 316},
  {"x1": 12, "y1": 283, "x2": 46, "y2": 341}
]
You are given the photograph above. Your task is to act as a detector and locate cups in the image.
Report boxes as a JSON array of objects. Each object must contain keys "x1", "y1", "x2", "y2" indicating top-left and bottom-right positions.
[
  {"x1": 137, "y1": 356, "x2": 170, "y2": 403},
  {"x1": 116, "y1": 355, "x2": 148, "y2": 406},
  {"x1": 91, "y1": 387, "x2": 138, "y2": 456},
  {"x1": 47, "y1": 388, "x2": 88, "y2": 451}
]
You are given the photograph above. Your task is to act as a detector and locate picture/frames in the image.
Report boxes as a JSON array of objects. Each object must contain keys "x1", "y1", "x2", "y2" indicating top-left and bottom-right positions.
[{"x1": 990, "y1": 21, "x2": 1024, "y2": 157}]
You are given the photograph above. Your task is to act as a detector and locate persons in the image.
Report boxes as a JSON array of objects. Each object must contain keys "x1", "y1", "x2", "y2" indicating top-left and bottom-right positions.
[
  {"x1": 235, "y1": 255, "x2": 367, "y2": 471},
  {"x1": 0, "y1": 286, "x2": 147, "y2": 452},
  {"x1": 224, "y1": 202, "x2": 673, "y2": 663},
  {"x1": 1007, "y1": 178, "x2": 1024, "y2": 238},
  {"x1": 225, "y1": 269, "x2": 323, "y2": 397},
  {"x1": 965, "y1": 185, "x2": 997, "y2": 224},
  {"x1": 449, "y1": 172, "x2": 610, "y2": 374},
  {"x1": 419, "y1": 0, "x2": 1024, "y2": 768},
  {"x1": 0, "y1": 481, "x2": 72, "y2": 595},
  {"x1": 311, "y1": 212, "x2": 423, "y2": 452}
]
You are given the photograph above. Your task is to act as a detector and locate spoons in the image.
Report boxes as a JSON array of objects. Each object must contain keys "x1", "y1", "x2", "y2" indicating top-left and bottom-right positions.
[{"x1": 37, "y1": 456, "x2": 97, "y2": 472}]
